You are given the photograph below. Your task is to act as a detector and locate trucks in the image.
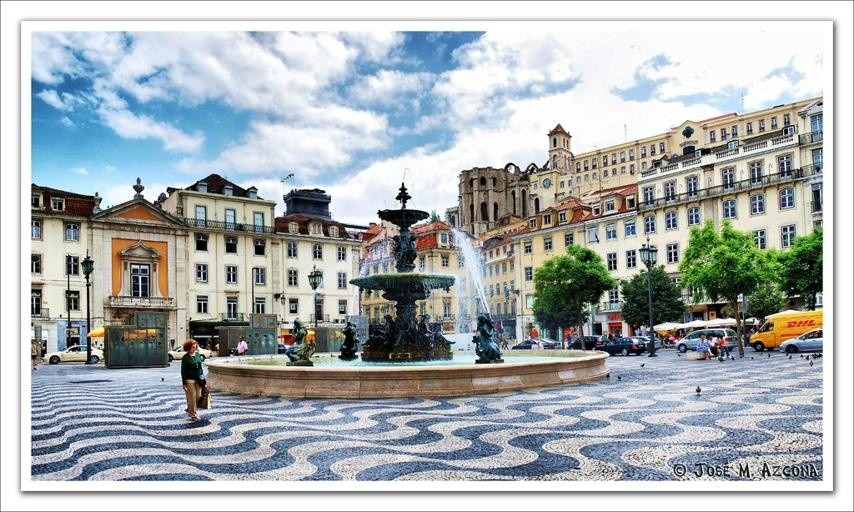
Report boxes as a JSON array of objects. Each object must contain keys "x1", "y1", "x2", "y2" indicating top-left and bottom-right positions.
[{"x1": 748, "y1": 310, "x2": 822, "y2": 351}]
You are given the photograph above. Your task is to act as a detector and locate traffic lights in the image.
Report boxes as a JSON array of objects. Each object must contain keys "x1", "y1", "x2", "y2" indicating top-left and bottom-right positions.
[
  {"x1": 490, "y1": 290, "x2": 494, "y2": 298},
  {"x1": 504, "y1": 289, "x2": 510, "y2": 303}
]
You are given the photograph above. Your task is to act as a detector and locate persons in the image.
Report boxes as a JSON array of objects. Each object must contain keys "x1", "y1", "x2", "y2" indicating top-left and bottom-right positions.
[
  {"x1": 31, "y1": 325, "x2": 250, "y2": 370},
  {"x1": 180, "y1": 339, "x2": 206, "y2": 421},
  {"x1": 501, "y1": 326, "x2": 819, "y2": 362},
  {"x1": 285, "y1": 313, "x2": 509, "y2": 362}
]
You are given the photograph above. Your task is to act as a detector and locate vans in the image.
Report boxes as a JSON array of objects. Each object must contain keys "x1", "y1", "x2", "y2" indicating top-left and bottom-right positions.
[{"x1": 672, "y1": 328, "x2": 738, "y2": 353}]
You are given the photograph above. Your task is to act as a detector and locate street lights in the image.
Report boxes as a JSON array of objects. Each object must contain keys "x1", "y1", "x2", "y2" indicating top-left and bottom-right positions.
[
  {"x1": 308, "y1": 264, "x2": 322, "y2": 327},
  {"x1": 638, "y1": 234, "x2": 658, "y2": 358},
  {"x1": 79, "y1": 248, "x2": 94, "y2": 365},
  {"x1": 251, "y1": 264, "x2": 265, "y2": 313},
  {"x1": 67, "y1": 253, "x2": 72, "y2": 346}
]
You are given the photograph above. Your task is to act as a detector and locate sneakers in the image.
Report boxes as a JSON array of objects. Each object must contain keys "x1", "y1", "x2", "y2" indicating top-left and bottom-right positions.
[{"x1": 191, "y1": 416, "x2": 201, "y2": 422}]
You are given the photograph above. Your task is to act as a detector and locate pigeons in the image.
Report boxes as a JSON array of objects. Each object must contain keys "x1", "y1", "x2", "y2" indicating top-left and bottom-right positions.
[
  {"x1": 716, "y1": 351, "x2": 823, "y2": 367},
  {"x1": 697, "y1": 385, "x2": 702, "y2": 396}
]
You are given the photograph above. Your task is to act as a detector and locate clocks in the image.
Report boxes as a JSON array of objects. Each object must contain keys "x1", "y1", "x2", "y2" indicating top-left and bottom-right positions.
[{"x1": 542, "y1": 178, "x2": 552, "y2": 189}]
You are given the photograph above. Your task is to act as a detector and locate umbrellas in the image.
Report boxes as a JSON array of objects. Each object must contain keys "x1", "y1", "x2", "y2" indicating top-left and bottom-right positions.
[{"x1": 645, "y1": 316, "x2": 760, "y2": 331}]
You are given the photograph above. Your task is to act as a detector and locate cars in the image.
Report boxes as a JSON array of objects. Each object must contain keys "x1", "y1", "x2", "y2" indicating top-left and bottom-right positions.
[
  {"x1": 567, "y1": 335, "x2": 664, "y2": 357},
  {"x1": 277, "y1": 343, "x2": 286, "y2": 353},
  {"x1": 42, "y1": 344, "x2": 103, "y2": 364},
  {"x1": 778, "y1": 329, "x2": 823, "y2": 353},
  {"x1": 510, "y1": 339, "x2": 562, "y2": 350},
  {"x1": 167, "y1": 345, "x2": 213, "y2": 362}
]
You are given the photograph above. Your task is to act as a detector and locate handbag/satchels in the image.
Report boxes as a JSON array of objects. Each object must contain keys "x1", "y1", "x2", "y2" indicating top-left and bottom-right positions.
[
  {"x1": 198, "y1": 375, "x2": 206, "y2": 387},
  {"x1": 197, "y1": 385, "x2": 213, "y2": 410}
]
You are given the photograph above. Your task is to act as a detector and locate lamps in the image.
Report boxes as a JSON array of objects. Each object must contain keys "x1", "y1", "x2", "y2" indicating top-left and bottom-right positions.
[
  {"x1": 274, "y1": 290, "x2": 286, "y2": 306},
  {"x1": 513, "y1": 287, "x2": 521, "y2": 299}
]
